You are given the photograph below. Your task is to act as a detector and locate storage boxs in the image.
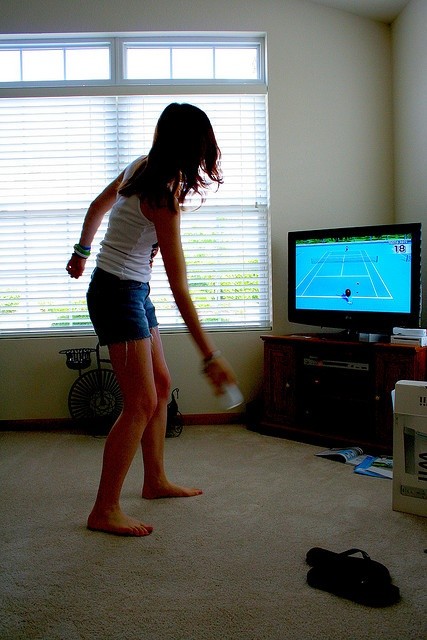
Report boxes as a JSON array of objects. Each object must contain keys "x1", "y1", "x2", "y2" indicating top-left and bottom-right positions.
[{"x1": 390, "y1": 380, "x2": 427, "y2": 517}]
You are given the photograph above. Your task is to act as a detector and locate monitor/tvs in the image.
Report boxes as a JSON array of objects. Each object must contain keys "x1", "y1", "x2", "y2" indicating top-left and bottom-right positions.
[{"x1": 288, "y1": 222, "x2": 422, "y2": 342}]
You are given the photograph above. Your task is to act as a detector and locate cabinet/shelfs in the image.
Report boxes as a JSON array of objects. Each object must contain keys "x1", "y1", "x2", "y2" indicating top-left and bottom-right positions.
[{"x1": 246, "y1": 332, "x2": 427, "y2": 450}]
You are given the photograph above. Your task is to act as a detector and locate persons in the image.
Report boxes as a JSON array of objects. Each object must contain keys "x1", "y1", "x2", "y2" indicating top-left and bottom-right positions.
[{"x1": 67, "y1": 102, "x2": 236, "y2": 537}]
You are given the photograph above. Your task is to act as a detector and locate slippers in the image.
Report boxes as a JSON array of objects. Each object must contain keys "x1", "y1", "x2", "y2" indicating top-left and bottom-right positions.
[
  {"x1": 307, "y1": 567, "x2": 400, "y2": 608},
  {"x1": 306, "y1": 547, "x2": 390, "y2": 581}
]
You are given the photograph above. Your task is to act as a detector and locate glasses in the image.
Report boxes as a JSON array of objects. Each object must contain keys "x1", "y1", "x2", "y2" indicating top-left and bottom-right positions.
[{"x1": 380, "y1": 454, "x2": 393, "y2": 461}]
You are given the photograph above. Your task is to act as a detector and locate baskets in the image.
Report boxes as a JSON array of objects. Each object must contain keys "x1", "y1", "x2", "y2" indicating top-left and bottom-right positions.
[{"x1": 59, "y1": 348, "x2": 96, "y2": 370}]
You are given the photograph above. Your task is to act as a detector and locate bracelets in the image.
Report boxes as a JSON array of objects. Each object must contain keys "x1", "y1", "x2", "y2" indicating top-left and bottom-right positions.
[
  {"x1": 71, "y1": 243, "x2": 91, "y2": 260},
  {"x1": 202, "y1": 349, "x2": 222, "y2": 366}
]
